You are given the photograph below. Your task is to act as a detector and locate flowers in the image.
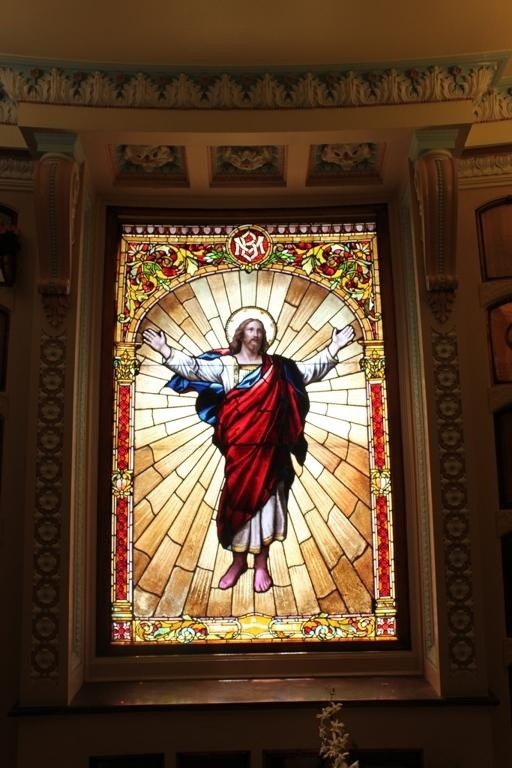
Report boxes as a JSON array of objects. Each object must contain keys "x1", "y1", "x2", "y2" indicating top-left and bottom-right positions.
[{"x1": 315, "y1": 702, "x2": 355, "y2": 768}]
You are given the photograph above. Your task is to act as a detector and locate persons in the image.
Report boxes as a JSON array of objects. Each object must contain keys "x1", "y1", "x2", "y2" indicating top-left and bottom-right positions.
[{"x1": 141, "y1": 318, "x2": 356, "y2": 592}]
